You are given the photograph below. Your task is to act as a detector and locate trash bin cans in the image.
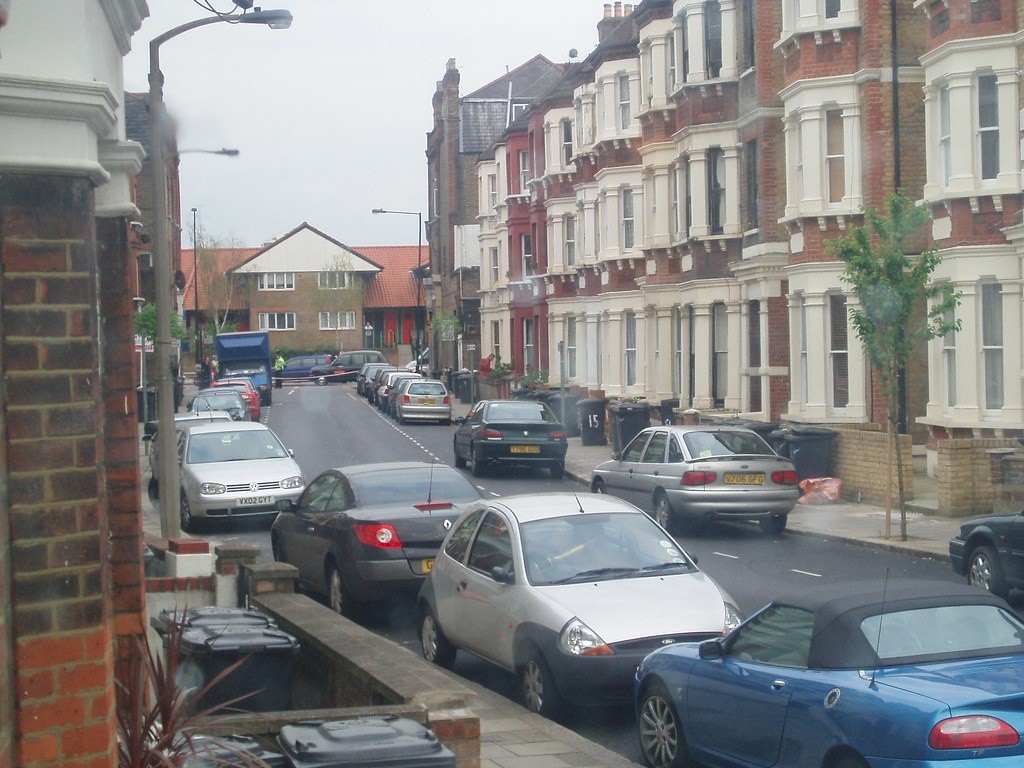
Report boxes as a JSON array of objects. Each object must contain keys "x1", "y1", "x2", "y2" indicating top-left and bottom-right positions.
[
  {"x1": 607, "y1": 404, "x2": 651, "y2": 452},
  {"x1": 276, "y1": 715, "x2": 455, "y2": 768},
  {"x1": 450, "y1": 372, "x2": 472, "y2": 403},
  {"x1": 150, "y1": 606, "x2": 302, "y2": 717},
  {"x1": 659, "y1": 397, "x2": 691, "y2": 426},
  {"x1": 709, "y1": 416, "x2": 840, "y2": 499},
  {"x1": 511, "y1": 389, "x2": 581, "y2": 439},
  {"x1": 170, "y1": 734, "x2": 284, "y2": 768},
  {"x1": 577, "y1": 398, "x2": 609, "y2": 445}
]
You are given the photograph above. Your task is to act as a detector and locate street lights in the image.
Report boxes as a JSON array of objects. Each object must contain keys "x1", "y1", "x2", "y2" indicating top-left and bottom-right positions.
[
  {"x1": 144, "y1": 1, "x2": 300, "y2": 537},
  {"x1": 191, "y1": 207, "x2": 202, "y2": 360},
  {"x1": 373, "y1": 208, "x2": 423, "y2": 373}
]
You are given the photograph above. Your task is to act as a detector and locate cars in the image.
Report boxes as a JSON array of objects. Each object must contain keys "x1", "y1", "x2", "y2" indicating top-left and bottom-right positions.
[
  {"x1": 398, "y1": 379, "x2": 456, "y2": 424},
  {"x1": 404, "y1": 347, "x2": 428, "y2": 373},
  {"x1": 946, "y1": 516, "x2": 1023, "y2": 589},
  {"x1": 184, "y1": 388, "x2": 253, "y2": 425},
  {"x1": 267, "y1": 460, "x2": 503, "y2": 618},
  {"x1": 210, "y1": 381, "x2": 265, "y2": 421},
  {"x1": 631, "y1": 577, "x2": 1024, "y2": 766},
  {"x1": 356, "y1": 363, "x2": 423, "y2": 415},
  {"x1": 588, "y1": 426, "x2": 800, "y2": 535},
  {"x1": 414, "y1": 492, "x2": 746, "y2": 718},
  {"x1": 145, "y1": 410, "x2": 240, "y2": 496},
  {"x1": 270, "y1": 355, "x2": 333, "y2": 384},
  {"x1": 175, "y1": 422, "x2": 304, "y2": 533},
  {"x1": 452, "y1": 400, "x2": 579, "y2": 477}
]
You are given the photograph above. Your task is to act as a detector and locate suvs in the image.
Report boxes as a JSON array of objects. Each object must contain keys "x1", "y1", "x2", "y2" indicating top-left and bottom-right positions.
[{"x1": 309, "y1": 350, "x2": 389, "y2": 386}]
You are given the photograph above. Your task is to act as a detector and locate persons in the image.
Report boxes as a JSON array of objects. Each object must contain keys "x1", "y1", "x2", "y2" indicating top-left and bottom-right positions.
[
  {"x1": 202, "y1": 354, "x2": 218, "y2": 389},
  {"x1": 480, "y1": 353, "x2": 496, "y2": 377},
  {"x1": 365, "y1": 322, "x2": 373, "y2": 336},
  {"x1": 274, "y1": 349, "x2": 286, "y2": 389}
]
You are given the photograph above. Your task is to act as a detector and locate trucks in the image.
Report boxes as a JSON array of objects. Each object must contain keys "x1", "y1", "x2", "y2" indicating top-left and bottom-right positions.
[{"x1": 213, "y1": 331, "x2": 275, "y2": 405}]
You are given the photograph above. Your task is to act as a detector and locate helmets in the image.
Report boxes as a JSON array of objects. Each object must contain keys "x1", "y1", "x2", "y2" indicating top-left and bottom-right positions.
[{"x1": 276, "y1": 349, "x2": 280, "y2": 355}]
